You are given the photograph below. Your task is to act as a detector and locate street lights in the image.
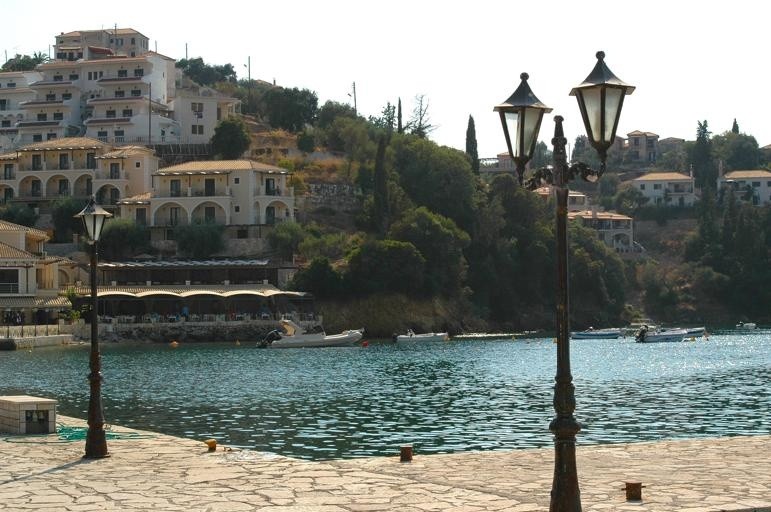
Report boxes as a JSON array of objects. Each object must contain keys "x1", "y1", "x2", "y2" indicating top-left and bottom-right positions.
[
  {"x1": 70, "y1": 195, "x2": 115, "y2": 459},
  {"x1": 492, "y1": 52, "x2": 636, "y2": 512}
]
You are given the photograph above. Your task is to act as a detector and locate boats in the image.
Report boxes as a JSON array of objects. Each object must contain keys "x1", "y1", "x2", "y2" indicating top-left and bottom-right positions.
[
  {"x1": 643, "y1": 325, "x2": 705, "y2": 342},
  {"x1": 398, "y1": 332, "x2": 449, "y2": 341},
  {"x1": 737, "y1": 320, "x2": 755, "y2": 330},
  {"x1": 270, "y1": 325, "x2": 366, "y2": 349},
  {"x1": 570, "y1": 329, "x2": 620, "y2": 339}
]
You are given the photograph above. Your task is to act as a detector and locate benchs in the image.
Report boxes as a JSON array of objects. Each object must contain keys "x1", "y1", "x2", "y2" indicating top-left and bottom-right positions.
[{"x1": 0, "y1": 395, "x2": 58, "y2": 434}]
